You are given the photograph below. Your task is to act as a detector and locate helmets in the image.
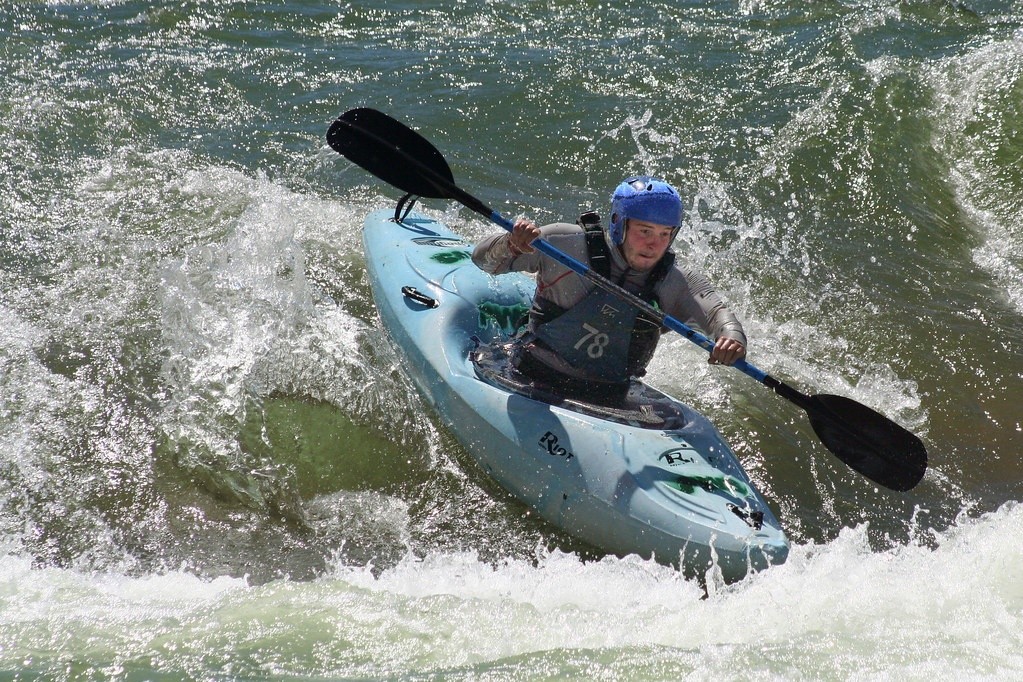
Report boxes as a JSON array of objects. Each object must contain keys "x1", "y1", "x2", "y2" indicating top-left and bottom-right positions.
[{"x1": 610, "y1": 176, "x2": 682, "y2": 250}]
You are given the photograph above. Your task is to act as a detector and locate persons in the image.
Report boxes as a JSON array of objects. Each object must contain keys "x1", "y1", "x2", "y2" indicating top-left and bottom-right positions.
[{"x1": 469, "y1": 176, "x2": 747, "y2": 407}]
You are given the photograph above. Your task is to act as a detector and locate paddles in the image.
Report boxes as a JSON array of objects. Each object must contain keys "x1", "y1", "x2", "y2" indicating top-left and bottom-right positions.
[{"x1": 326, "y1": 107, "x2": 928, "y2": 492}]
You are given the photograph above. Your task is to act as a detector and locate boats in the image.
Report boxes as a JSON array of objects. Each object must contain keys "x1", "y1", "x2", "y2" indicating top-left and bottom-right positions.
[{"x1": 361, "y1": 205, "x2": 791, "y2": 587}]
